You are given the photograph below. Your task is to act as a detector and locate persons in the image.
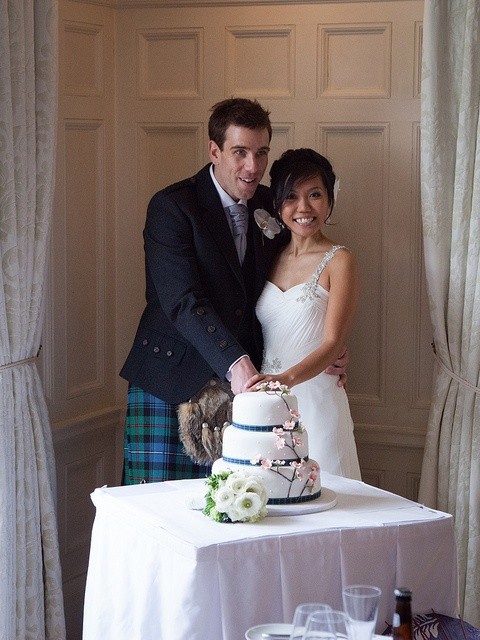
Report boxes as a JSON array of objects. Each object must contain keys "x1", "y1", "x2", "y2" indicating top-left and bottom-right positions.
[
  {"x1": 121, "y1": 99, "x2": 290, "y2": 486},
  {"x1": 253, "y1": 148, "x2": 363, "y2": 482}
]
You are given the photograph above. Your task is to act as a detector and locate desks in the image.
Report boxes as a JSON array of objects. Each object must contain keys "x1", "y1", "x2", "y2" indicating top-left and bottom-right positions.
[{"x1": 80, "y1": 461, "x2": 460, "y2": 637}]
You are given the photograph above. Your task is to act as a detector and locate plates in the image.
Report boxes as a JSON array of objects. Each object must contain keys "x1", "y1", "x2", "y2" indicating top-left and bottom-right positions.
[{"x1": 245, "y1": 623, "x2": 292, "y2": 640}]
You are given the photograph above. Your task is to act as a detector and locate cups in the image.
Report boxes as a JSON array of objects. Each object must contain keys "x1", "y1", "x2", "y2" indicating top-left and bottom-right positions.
[
  {"x1": 302, "y1": 611, "x2": 352, "y2": 640},
  {"x1": 342, "y1": 584, "x2": 382, "y2": 640},
  {"x1": 290, "y1": 603, "x2": 333, "y2": 640}
]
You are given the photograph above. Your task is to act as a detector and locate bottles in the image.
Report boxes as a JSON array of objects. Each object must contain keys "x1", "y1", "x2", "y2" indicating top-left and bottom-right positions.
[{"x1": 391, "y1": 587, "x2": 414, "y2": 640}]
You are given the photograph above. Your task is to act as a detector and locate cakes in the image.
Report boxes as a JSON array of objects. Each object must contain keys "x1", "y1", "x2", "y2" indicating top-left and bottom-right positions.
[{"x1": 211, "y1": 380, "x2": 321, "y2": 504}]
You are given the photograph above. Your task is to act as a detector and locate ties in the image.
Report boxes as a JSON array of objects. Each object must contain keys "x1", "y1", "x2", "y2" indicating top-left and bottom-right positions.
[{"x1": 228, "y1": 203, "x2": 248, "y2": 267}]
